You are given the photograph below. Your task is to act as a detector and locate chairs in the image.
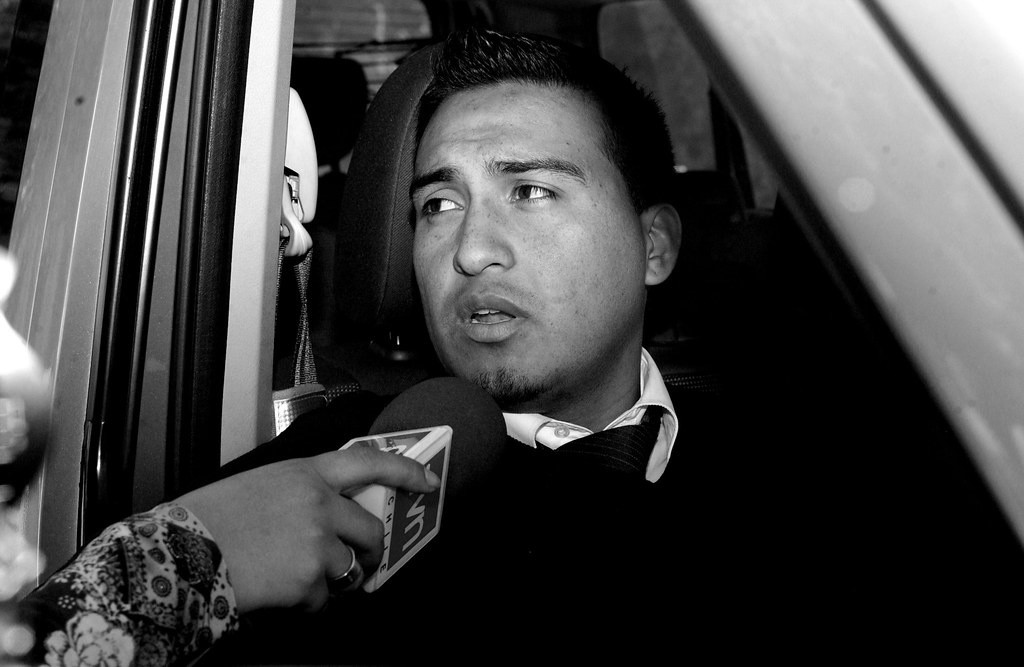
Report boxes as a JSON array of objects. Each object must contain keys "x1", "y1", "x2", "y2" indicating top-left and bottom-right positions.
[
  {"x1": 313, "y1": 40, "x2": 446, "y2": 397},
  {"x1": 291, "y1": 55, "x2": 370, "y2": 231}
]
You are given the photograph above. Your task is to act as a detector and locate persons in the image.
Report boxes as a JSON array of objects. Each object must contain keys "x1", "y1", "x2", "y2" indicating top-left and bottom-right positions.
[
  {"x1": 178, "y1": 22, "x2": 899, "y2": 667},
  {"x1": 0, "y1": 246, "x2": 440, "y2": 667}
]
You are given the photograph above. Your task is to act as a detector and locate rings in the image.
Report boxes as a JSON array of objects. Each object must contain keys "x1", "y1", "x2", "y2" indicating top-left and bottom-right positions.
[{"x1": 333, "y1": 545, "x2": 362, "y2": 590}]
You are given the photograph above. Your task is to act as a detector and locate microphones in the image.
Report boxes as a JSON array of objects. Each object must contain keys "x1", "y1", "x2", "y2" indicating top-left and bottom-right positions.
[{"x1": 338, "y1": 376, "x2": 508, "y2": 593}]
